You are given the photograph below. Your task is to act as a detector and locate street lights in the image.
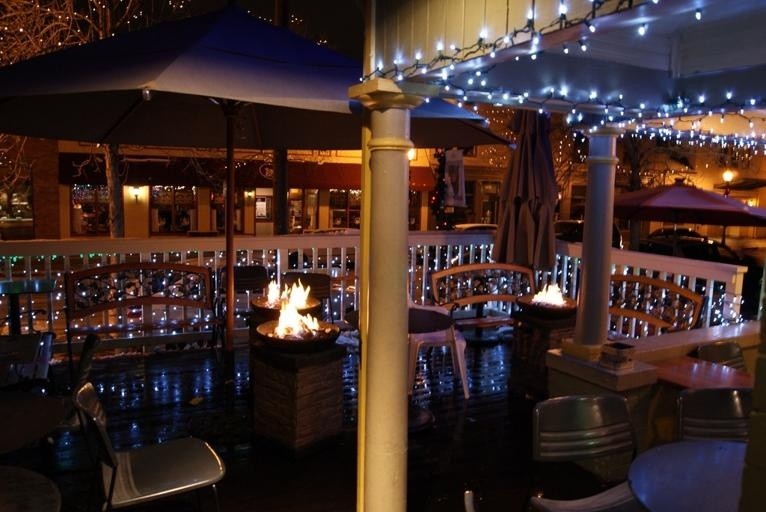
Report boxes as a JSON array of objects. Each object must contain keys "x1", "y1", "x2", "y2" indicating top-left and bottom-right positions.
[{"x1": 718, "y1": 166, "x2": 737, "y2": 245}]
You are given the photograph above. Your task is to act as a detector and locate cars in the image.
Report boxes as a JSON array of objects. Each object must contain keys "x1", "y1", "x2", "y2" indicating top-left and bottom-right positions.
[
  {"x1": 449, "y1": 221, "x2": 499, "y2": 231},
  {"x1": 647, "y1": 224, "x2": 711, "y2": 240},
  {"x1": 287, "y1": 227, "x2": 361, "y2": 270},
  {"x1": 104, "y1": 257, "x2": 355, "y2": 328}
]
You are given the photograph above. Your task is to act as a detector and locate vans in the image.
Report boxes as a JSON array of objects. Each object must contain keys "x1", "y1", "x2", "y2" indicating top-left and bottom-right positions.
[{"x1": 553, "y1": 216, "x2": 627, "y2": 264}]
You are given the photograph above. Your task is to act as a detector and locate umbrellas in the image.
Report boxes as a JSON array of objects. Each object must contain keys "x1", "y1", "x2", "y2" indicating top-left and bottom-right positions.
[
  {"x1": 491, "y1": 109, "x2": 560, "y2": 274},
  {"x1": 0, "y1": 0, "x2": 518, "y2": 444},
  {"x1": 572, "y1": 177, "x2": 766, "y2": 260}
]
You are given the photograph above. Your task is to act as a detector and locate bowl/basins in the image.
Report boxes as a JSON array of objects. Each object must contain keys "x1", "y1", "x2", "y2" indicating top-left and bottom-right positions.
[
  {"x1": 257, "y1": 319, "x2": 341, "y2": 352},
  {"x1": 517, "y1": 294, "x2": 579, "y2": 320},
  {"x1": 251, "y1": 296, "x2": 322, "y2": 317}
]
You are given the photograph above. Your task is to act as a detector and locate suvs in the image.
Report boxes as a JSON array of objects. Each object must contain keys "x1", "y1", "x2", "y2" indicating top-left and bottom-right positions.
[{"x1": 636, "y1": 233, "x2": 760, "y2": 321}]
